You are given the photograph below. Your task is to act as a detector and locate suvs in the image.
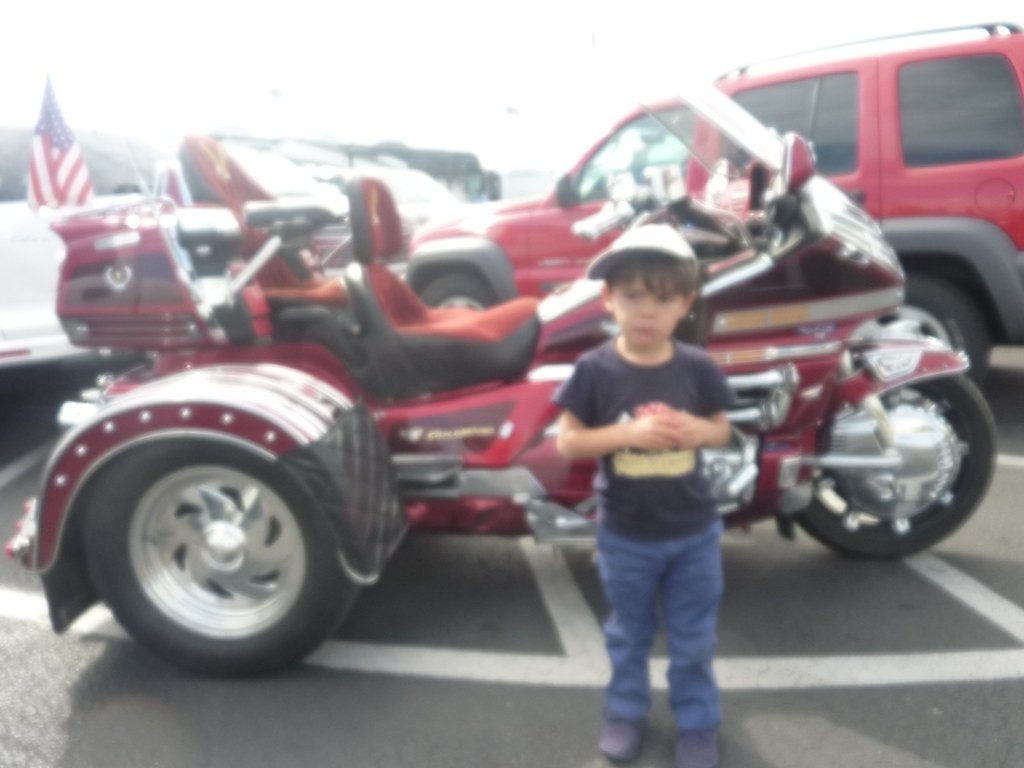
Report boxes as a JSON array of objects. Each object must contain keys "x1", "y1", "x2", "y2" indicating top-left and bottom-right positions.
[{"x1": 403, "y1": 20, "x2": 1024, "y2": 399}]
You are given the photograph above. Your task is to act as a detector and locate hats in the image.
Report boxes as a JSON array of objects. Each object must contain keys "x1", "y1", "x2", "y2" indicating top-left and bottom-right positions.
[{"x1": 586, "y1": 224, "x2": 697, "y2": 280}]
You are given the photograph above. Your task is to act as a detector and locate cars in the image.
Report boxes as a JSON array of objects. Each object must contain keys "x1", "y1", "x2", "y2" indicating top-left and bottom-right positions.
[{"x1": 0, "y1": 130, "x2": 507, "y2": 370}]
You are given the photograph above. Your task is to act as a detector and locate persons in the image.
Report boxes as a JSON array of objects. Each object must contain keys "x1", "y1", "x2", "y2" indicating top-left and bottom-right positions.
[{"x1": 553, "y1": 222, "x2": 739, "y2": 768}]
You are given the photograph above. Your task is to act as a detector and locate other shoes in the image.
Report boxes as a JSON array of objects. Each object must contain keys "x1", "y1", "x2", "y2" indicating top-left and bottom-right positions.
[
  {"x1": 600, "y1": 715, "x2": 643, "y2": 761},
  {"x1": 674, "y1": 728, "x2": 719, "y2": 768}
]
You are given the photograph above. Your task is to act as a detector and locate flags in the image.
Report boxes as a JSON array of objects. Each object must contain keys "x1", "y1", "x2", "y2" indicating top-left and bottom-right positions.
[{"x1": 27, "y1": 84, "x2": 91, "y2": 208}]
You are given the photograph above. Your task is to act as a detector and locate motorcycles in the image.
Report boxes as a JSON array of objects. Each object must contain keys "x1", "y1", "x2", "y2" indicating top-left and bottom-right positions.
[{"x1": 5, "y1": 73, "x2": 996, "y2": 678}]
